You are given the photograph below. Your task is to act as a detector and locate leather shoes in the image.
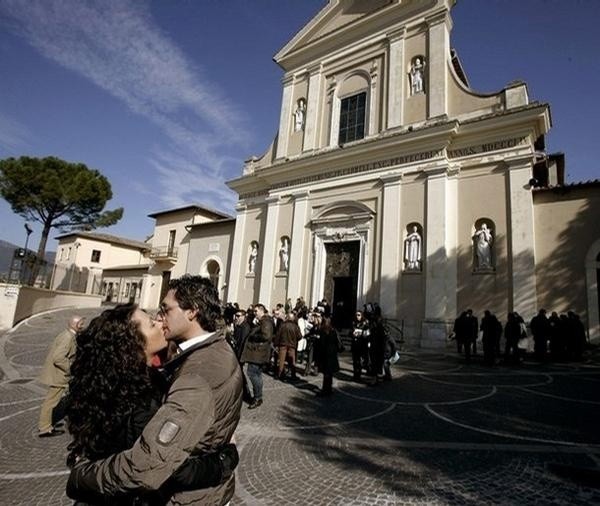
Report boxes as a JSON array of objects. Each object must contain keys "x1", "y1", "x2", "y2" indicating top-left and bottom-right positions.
[
  {"x1": 39, "y1": 429, "x2": 65, "y2": 437},
  {"x1": 52, "y1": 423, "x2": 64, "y2": 428},
  {"x1": 248, "y1": 399, "x2": 263, "y2": 410}
]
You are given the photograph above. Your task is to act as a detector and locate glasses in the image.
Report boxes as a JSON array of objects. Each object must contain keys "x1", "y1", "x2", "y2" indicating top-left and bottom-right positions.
[{"x1": 159, "y1": 303, "x2": 182, "y2": 316}]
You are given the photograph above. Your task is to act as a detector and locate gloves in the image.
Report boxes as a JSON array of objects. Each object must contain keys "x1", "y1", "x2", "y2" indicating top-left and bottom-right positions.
[{"x1": 218, "y1": 442, "x2": 239, "y2": 472}]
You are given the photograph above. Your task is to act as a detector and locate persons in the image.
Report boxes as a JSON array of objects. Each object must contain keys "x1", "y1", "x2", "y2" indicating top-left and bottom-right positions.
[
  {"x1": 221, "y1": 298, "x2": 395, "y2": 409},
  {"x1": 474, "y1": 222, "x2": 492, "y2": 267},
  {"x1": 453, "y1": 308, "x2": 586, "y2": 370},
  {"x1": 403, "y1": 226, "x2": 421, "y2": 270},
  {"x1": 249, "y1": 244, "x2": 257, "y2": 271},
  {"x1": 280, "y1": 238, "x2": 289, "y2": 270},
  {"x1": 66, "y1": 273, "x2": 248, "y2": 505},
  {"x1": 37, "y1": 313, "x2": 85, "y2": 436},
  {"x1": 295, "y1": 100, "x2": 304, "y2": 128},
  {"x1": 67, "y1": 302, "x2": 241, "y2": 505},
  {"x1": 411, "y1": 58, "x2": 424, "y2": 93}
]
